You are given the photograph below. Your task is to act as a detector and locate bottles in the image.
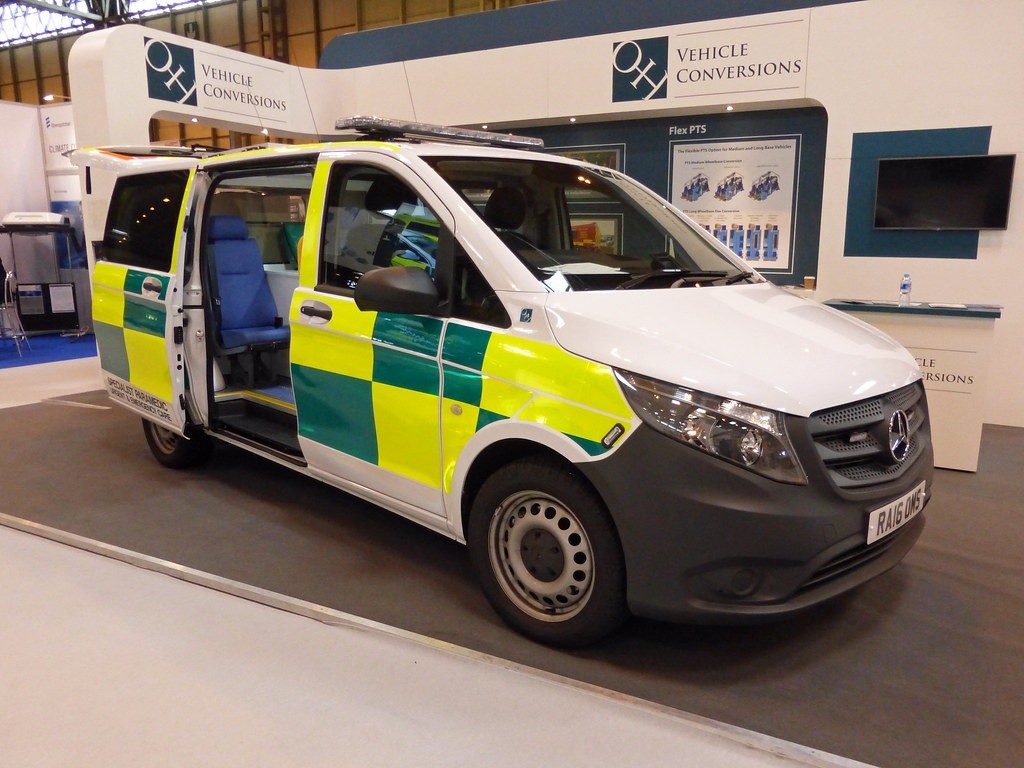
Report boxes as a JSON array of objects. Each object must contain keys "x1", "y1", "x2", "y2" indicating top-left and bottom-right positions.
[{"x1": 899, "y1": 273, "x2": 912, "y2": 307}]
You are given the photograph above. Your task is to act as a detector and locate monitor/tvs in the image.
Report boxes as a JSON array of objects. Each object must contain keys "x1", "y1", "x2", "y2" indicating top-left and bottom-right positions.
[{"x1": 873, "y1": 154, "x2": 1017, "y2": 231}]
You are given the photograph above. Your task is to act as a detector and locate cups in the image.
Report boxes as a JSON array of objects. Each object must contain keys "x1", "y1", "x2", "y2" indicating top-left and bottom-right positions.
[{"x1": 804, "y1": 277, "x2": 815, "y2": 288}]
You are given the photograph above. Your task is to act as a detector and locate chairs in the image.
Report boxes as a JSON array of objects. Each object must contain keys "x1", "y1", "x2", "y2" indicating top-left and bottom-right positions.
[
  {"x1": 206, "y1": 216, "x2": 290, "y2": 391},
  {"x1": 467, "y1": 186, "x2": 542, "y2": 317},
  {"x1": 1, "y1": 270, "x2": 34, "y2": 357},
  {"x1": 365, "y1": 177, "x2": 418, "y2": 213}
]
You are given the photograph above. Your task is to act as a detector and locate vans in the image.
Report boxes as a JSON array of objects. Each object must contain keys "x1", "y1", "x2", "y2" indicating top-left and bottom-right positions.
[{"x1": 69, "y1": 116, "x2": 934, "y2": 651}]
